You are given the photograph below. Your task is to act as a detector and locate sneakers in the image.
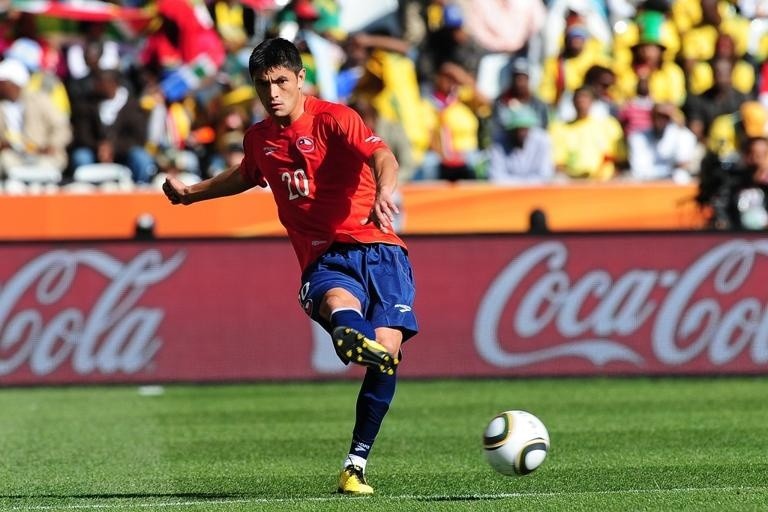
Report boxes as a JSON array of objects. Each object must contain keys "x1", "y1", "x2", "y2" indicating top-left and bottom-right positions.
[
  {"x1": 331, "y1": 325, "x2": 400, "y2": 376},
  {"x1": 338, "y1": 465, "x2": 375, "y2": 495}
]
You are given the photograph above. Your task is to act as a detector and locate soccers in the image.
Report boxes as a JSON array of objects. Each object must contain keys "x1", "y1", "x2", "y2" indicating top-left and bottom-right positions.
[{"x1": 485, "y1": 411, "x2": 550, "y2": 477}]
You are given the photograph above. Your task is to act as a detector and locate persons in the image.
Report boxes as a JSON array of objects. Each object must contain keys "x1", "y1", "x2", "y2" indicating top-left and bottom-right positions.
[
  {"x1": 0, "y1": 0, "x2": 767, "y2": 234},
  {"x1": 163, "y1": 39, "x2": 418, "y2": 496}
]
